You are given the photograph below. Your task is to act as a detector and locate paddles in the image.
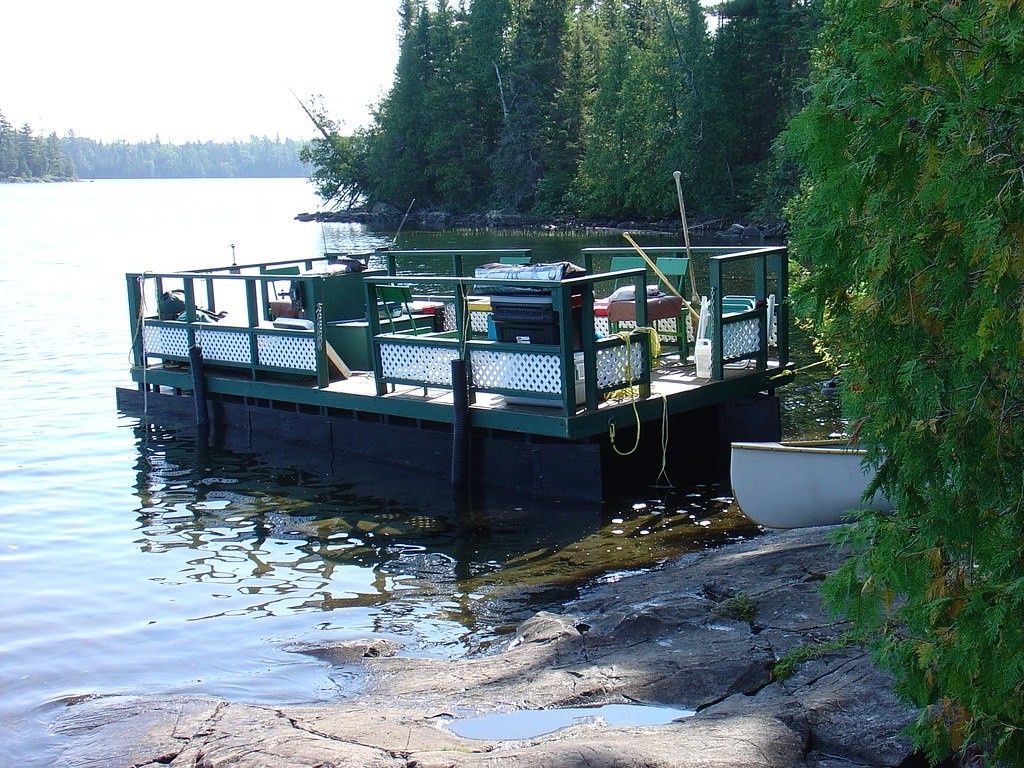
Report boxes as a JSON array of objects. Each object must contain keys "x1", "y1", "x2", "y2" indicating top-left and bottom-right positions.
[{"x1": 672, "y1": 171, "x2": 701, "y2": 343}]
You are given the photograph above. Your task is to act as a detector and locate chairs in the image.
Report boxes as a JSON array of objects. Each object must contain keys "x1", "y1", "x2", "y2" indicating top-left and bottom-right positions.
[
  {"x1": 597, "y1": 256, "x2": 690, "y2": 363},
  {"x1": 379, "y1": 285, "x2": 461, "y2": 395},
  {"x1": 262, "y1": 266, "x2": 300, "y2": 319}
]
